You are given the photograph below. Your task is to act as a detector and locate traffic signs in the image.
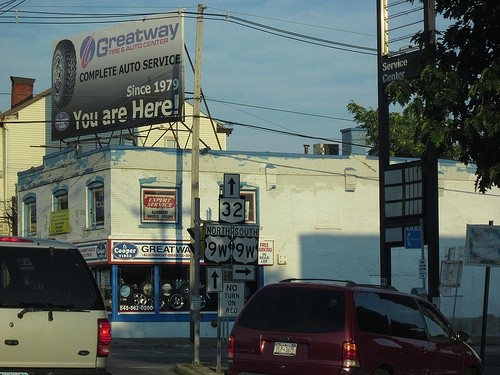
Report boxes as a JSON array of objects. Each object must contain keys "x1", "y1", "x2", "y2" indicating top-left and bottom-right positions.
[{"x1": 201, "y1": 173, "x2": 259, "y2": 317}]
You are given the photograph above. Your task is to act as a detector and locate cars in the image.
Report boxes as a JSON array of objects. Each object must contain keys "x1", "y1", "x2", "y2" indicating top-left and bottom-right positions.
[
  {"x1": 227, "y1": 278, "x2": 485, "y2": 375},
  {"x1": 0, "y1": 235, "x2": 111, "y2": 375}
]
